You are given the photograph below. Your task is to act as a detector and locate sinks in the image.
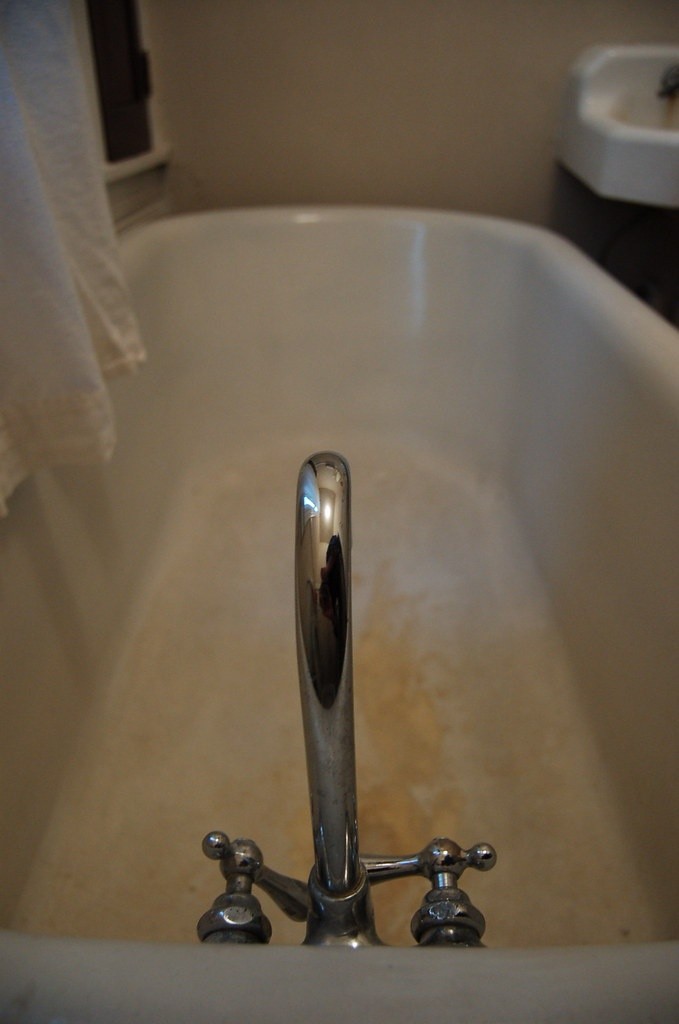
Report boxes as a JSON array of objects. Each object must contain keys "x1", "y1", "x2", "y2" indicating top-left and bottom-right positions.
[{"x1": 554, "y1": 40, "x2": 679, "y2": 207}]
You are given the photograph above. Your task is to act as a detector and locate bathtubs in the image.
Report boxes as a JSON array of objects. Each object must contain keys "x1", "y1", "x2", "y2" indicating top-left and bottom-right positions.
[{"x1": 0, "y1": 201, "x2": 679, "y2": 1022}]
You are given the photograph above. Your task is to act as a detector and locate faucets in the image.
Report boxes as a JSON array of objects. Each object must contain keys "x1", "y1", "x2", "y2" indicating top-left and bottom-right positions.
[{"x1": 195, "y1": 451, "x2": 498, "y2": 953}]
